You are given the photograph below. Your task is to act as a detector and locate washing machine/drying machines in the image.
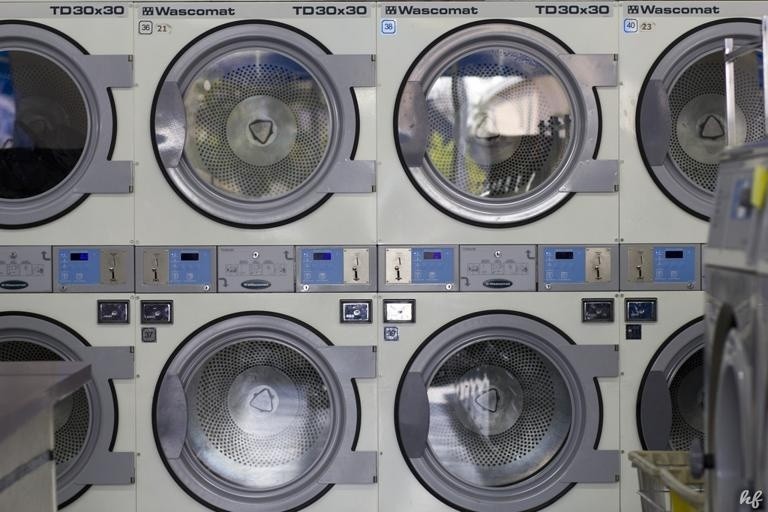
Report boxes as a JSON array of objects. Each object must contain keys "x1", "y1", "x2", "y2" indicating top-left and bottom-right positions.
[{"x1": 0, "y1": 1, "x2": 767, "y2": 512}]
